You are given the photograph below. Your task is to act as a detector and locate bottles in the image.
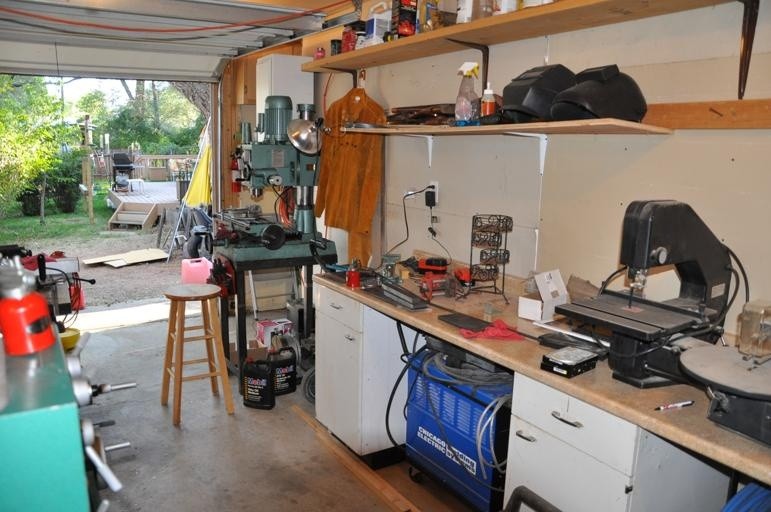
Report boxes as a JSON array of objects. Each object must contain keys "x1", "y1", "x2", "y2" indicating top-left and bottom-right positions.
[{"x1": 481, "y1": 82, "x2": 496, "y2": 116}]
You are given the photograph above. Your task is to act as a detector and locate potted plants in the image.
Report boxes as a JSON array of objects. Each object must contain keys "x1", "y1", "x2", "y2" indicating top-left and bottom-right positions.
[{"x1": 17, "y1": 175, "x2": 81, "y2": 216}]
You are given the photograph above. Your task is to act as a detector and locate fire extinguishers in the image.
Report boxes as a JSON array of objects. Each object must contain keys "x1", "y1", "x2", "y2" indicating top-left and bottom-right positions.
[{"x1": 230, "y1": 154, "x2": 241, "y2": 192}]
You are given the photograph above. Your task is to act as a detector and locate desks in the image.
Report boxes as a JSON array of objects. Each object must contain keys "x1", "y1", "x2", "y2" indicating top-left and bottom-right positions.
[{"x1": 310, "y1": 261, "x2": 771, "y2": 512}]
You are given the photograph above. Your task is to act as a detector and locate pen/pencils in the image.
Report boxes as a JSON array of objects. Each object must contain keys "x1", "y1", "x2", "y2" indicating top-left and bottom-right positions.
[{"x1": 654, "y1": 401, "x2": 695, "y2": 410}]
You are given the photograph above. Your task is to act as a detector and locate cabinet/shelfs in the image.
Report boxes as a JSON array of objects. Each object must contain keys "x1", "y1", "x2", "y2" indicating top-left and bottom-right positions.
[{"x1": 301, "y1": 2, "x2": 731, "y2": 136}]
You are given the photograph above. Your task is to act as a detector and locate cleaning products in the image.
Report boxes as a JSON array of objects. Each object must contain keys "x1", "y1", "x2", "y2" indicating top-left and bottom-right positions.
[{"x1": 454, "y1": 62, "x2": 480, "y2": 123}]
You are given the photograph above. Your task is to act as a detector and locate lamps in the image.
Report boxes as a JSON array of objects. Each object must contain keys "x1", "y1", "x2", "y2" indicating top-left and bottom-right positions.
[{"x1": 287, "y1": 115, "x2": 388, "y2": 156}]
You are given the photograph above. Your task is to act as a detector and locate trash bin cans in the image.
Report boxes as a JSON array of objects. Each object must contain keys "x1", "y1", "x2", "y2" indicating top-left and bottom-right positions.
[{"x1": 177, "y1": 181, "x2": 190, "y2": 201}]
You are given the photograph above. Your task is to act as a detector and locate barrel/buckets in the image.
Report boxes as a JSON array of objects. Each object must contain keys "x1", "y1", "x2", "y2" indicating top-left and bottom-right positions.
[
  {"x1": 128, "y1": 178, "x2": 143, "y2": 193},
  {"x1": 181, "y1": 257, "x2": 213, "y2": 284},
  {"x1": 268, "y1": 347, "x2": 296, "y2": 395},
  {"x1": 242, "y1": 356, "x2": 276, "y2": 409}
]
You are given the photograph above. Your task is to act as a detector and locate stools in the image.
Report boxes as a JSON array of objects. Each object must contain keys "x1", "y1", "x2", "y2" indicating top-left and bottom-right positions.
[{"x1": 161, "y1": 283, "x2": 235, "y2": 427}]
